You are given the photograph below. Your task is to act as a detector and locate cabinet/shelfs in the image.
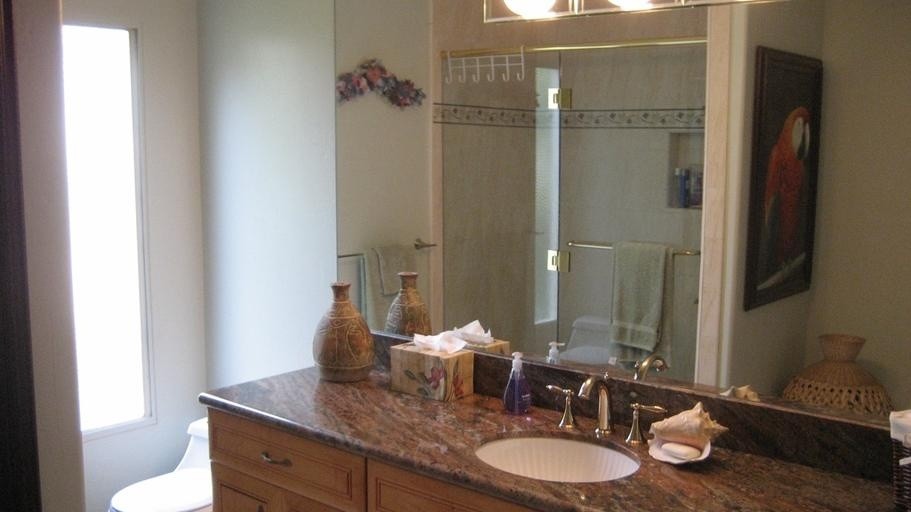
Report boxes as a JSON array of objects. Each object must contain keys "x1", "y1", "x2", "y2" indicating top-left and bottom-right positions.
[{"x1": 200, "y1": 354, "x2": 910, "y2": 511}]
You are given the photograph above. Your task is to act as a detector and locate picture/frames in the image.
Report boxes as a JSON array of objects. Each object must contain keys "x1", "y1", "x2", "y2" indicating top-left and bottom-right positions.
[{"x1": 744, "y1": 44, "x2": 821, "y2": 310}]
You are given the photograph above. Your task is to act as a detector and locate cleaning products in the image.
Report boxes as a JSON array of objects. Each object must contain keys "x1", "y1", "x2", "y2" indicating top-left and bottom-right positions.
[{"x1": 504, "y1": 351, "x2": 531, "y2": 415}]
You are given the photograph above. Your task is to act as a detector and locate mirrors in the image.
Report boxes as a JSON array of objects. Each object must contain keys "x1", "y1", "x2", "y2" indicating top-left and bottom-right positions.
[{"x1": 333, "y1": 1, "x2": 911, "y2": 434}]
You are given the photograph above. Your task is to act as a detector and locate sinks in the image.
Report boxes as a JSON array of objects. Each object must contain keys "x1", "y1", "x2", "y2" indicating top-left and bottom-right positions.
[{"x1": 474, "y1": 428, "x2": 645, "y2": 483}]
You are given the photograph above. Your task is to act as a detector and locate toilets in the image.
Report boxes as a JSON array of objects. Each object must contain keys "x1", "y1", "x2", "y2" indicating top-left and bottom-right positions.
[{"x1": 559, "y1": 316, "x2": 624, "y2": 368}]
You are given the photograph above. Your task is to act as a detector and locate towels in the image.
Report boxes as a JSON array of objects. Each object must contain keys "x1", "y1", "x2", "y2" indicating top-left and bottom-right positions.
[
  {"x1": 371, "y1": 243, "x2": 407, "y2": 296},
  {"x1": 360, "y1": 245, "x2": 417, "y2": 331},
  {"x1": 607, "y1": 240, "x2": 675, "y2": 380}
]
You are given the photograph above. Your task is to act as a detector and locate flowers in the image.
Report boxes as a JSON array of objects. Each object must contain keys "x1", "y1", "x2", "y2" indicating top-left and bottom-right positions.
[{"x1": 334, "y1": 60, "x2": 427, "y2": 110}]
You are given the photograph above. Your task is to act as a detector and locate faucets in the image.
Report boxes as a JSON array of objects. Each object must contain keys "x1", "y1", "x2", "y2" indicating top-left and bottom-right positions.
[
  {"x1": 577, "y1": 375, "x2": 615, "y2": 434},
  {"x1": 631, "y1": 354, "x2": 669, "y2": 382}
]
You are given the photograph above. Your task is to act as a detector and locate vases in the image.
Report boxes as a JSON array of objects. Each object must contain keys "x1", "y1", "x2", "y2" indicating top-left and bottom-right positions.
[
  {"x1": 778, "y1": 333, "x2": 896, "y2": 415},
  {"x1": 383, "y1": 272, "x2": 432, "y2": 337},
  {"x1": 314, "y1": 282, "x2": 375, "y2": 383}
]
